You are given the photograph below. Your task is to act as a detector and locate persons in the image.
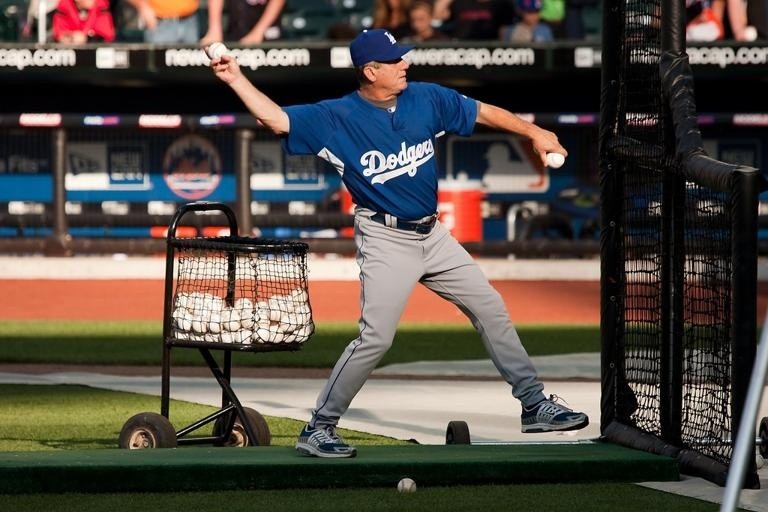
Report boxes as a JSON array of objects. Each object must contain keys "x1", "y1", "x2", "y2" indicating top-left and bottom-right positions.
[
  {"x1": 0, "y1": 0, "x2": 767, "y2": 48},
  {"x1": 203, "y1": 25, "x2": 593, "y2": 460}
]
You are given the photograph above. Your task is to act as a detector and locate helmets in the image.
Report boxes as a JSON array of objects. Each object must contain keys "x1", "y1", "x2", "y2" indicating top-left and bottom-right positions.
[{"x1": 516, "y1": 0, "x2": 544, "y2": 14}]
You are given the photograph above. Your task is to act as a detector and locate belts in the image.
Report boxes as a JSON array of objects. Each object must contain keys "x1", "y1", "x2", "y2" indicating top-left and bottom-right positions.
[
  {"x1": 370, "y1": 212, "x2": 442, "y2": 235},
  {"x1": 154, "y1": 8, "x2": 201, "y2": 20}
]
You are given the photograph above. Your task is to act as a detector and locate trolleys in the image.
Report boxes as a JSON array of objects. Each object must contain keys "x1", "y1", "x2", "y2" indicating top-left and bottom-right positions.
[{"x1": 117, "y1": 203, "x2": 316, "y2": 449}]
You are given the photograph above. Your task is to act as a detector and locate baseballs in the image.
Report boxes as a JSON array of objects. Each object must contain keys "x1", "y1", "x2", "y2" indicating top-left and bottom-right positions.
[
  {"x1": 173, "y1": 288, "x2": 312, "y2": 345},
  {"x1": 397, "y1": 478, "x2": 417, "y2": 493},
  {"x1": 207, "y1": 42, "x2": 226, "y2": 59},
  {"x1": 547, "y1": 153, "x2": 565, "y2": 169}
]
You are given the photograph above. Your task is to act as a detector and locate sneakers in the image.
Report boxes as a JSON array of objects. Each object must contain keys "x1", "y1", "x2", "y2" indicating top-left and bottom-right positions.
[
  {"x1": 294, "y1": 420, "x2": 358, "y2": 459},
  {"x1": 520, "y1": 394, "x2": 590, "y2": 435}
]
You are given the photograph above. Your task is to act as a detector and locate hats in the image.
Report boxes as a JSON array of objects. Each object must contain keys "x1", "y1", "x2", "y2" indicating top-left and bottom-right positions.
[{"x1": 346, "y1": 27, "x2": 417, "y2": 68}]
